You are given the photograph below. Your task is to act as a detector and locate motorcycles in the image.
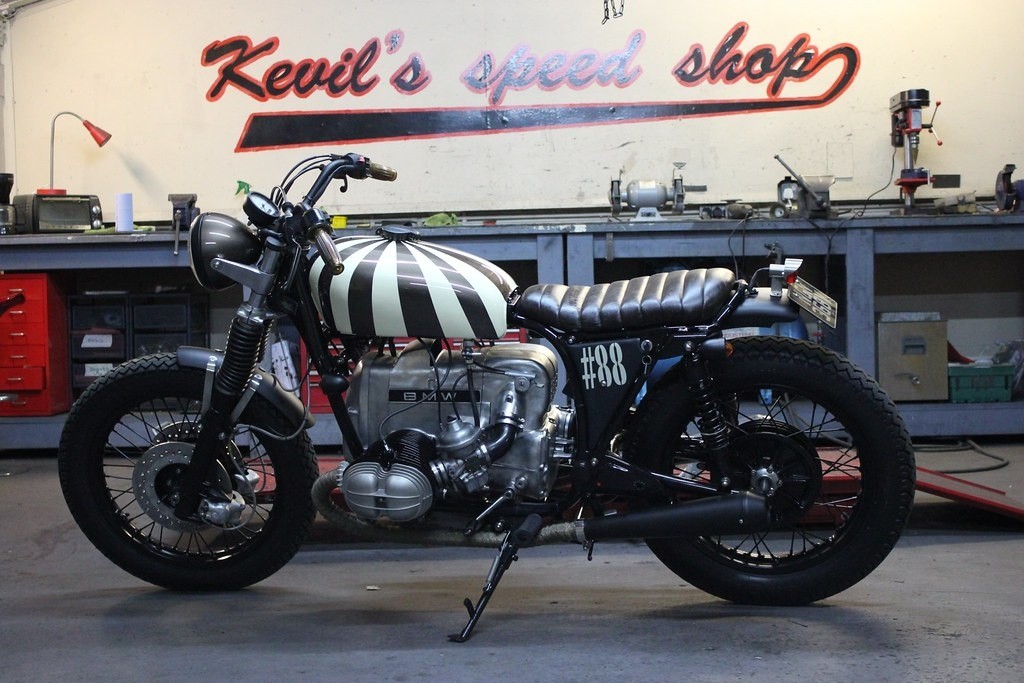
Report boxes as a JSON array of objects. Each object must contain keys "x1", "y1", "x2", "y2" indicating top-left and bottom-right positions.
[{"x1": 58, "y1": 152, "x2": 916, "y2": 645}]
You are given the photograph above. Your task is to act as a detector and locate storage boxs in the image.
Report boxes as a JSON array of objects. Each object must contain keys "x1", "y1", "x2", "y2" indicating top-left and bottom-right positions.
[
  {"x1": 948, "y1": 366, "x2": 1015, "y2": 403},
  {"x1": 0, "y1": 272, "x2": 72, "y2": 417},
  {"x1": 876, "y1": 311, "x2": 948, "y2": 401}
]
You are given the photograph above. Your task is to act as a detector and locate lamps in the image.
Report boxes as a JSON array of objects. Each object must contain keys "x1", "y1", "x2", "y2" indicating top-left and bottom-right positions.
[{"x1": 36, "y1": 113, "x2": 112, "y2": 195}]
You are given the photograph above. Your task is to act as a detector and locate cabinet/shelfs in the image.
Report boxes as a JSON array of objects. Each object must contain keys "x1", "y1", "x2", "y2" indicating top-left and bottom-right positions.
[
  {"x1": 0, "y1": 231, "x2": 249, "y2": 452},
  {"x1": 250, "y1": 212, "x2": 1024, "y2": 462},
  {"x1": 66, "y1": 289, "x2": 209, "y2": 411}
]
